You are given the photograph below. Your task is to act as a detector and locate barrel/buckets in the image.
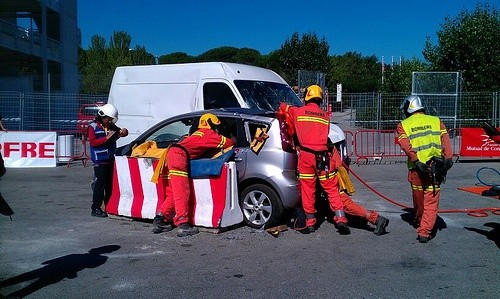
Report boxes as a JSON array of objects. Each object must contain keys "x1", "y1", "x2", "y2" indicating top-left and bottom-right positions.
[{"x1": 58, "y1": 134, "x2": 75, "y2": 161}]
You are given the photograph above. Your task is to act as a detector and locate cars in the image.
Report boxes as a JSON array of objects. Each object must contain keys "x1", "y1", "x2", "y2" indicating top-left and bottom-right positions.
[{"x1": 92, "y1": 107, "x2": 317, "y2": 230}]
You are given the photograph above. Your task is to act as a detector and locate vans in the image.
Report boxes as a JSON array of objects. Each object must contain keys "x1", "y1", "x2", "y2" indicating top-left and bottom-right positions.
[
  {"x1": 107, "y1": 62, "x2": 349, "y2": 173},
  {"x1": 76, "y1": 101, "x2": 107, "y2": 141}
]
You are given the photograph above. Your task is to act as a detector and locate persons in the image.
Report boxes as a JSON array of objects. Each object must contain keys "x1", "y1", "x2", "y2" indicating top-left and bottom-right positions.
[
  {"x1": 0, "y1": 114, "x2": 7, "y2": 131},
  {"x1": 0, "y1": 152, "x2": 14, "y2": 216},
  {"x1": 482, "y1": 122, "x2": 500, "y2": 143},
  {"x1": 88, "y1": 104, "x2": 128, "y2": 216},
  {"x1": 152, "y1": 113, "x2": 236, "y2": 236},
  {"x1": 279, "y1": 84, "x2": 350, "y2": 234},
  {"x1": 396, "y1": 95, "x2": 452, "y2": 243},
  {"x1": 339, "y1": 188, "x2": 390, "y2": 235}
]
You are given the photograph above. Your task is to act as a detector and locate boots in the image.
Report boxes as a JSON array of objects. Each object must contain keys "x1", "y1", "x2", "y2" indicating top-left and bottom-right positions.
[
  {"x1": 177, "y1": 224, "x2": 198, "y2": 236},
  {"x1": 153, "y1": 218, "x2": 173, "y2": 232}
]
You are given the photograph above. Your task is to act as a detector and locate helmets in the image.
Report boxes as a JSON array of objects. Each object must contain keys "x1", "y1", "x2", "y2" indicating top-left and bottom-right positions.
[
  {"x1": 101, "y1": 104, "x2": 118, "y2": 118},
  {"x1": 304, "y1": 85, "x2": 323, "y2": 101},
  {"x1": 400, "y1": 96, "x2": 425, "y2": 114},
  {"x1": 198, "y1": 113, "x2": 221, "y2": 129}
]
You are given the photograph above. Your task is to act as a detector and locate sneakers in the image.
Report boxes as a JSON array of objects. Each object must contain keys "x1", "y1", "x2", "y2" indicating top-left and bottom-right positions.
[{"x1": 92, "y1": 209, "x2": 105, "y2": 217}]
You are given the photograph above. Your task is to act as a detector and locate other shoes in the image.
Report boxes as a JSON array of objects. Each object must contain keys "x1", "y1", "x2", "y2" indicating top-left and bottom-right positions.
[
  {"x1": 420, "y1": 236, "x2": 427, "y2": 243},
  {"x1": 308, "y1": 227, "x2": 314, "y2": 232},
  {"x1": 338, "y1": 222, "x2": 350, "y2": 234},
  {"x1": 374, "y1": 215, "x2": 389, "y2": 235}
]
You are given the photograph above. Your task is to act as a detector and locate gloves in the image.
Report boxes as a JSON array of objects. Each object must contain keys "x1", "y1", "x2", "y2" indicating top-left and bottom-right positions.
[
  {"x1": 415, "y1": 162, "x2": 427, "y2": 174},
  {"x1": 445, "y1": 158, "x2": 453, "y2": 170}
]
